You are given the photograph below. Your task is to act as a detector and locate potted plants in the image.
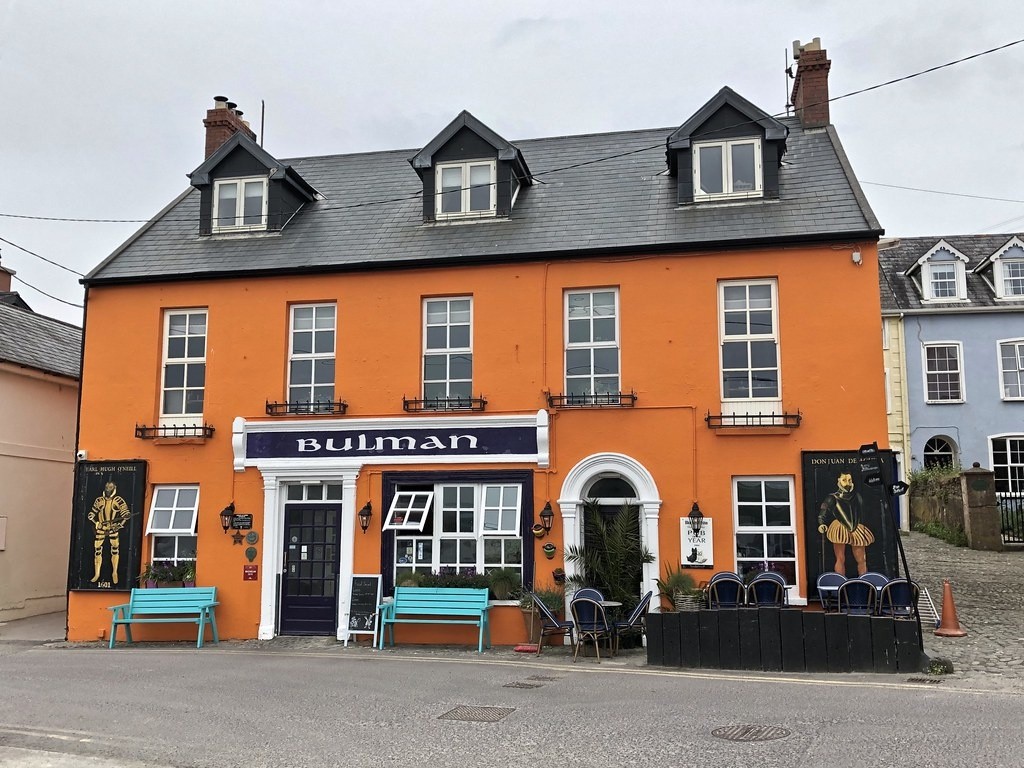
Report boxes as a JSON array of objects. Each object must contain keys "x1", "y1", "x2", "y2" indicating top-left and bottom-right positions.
[
  {"x1": 532, "y1": 524, "x2": 545, "y2": 537},
  {"x1": 520, "y1": 588, "x2": 563, "y2": 645},
  {"x1": 544, "y1": 544, "x2": 556, "y2": 559},
  {"x1": 552, "y1": 568, "x2": 565, "y2": 585},
  {"x1": 396, "y1": 571, "x2": 421, "y2": 587},
  {"x1": 492, "y1": 569, "x2": 520, "y2": 600}
]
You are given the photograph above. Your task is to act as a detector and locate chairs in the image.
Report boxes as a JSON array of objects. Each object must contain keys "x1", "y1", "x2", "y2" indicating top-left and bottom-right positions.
[
  {"x1": 530, "y1": 593, "x2": 575, "y2": 657},
  {"x1": 571, "y1": 596, "x2": 613, "y2": 664},
  {"x1": 879, "y1": 578, "x2": 920, "y2": 620},
  {"x1": 838, "y1": 578, "x2": 877, "y2": 616},
  {"x1": 573, "y1": 589, "x2": 604, "y2": 601},
  {"x1": 708, "y1": 579, "x2": 745, "y2": 607},
  {"x1": 747, "y1": 577, "x2": 784, "y2": 608},
  {"x1": 612, "y1": 591, "x2": 652, "y2": 656},
  {"x1": 818, "y1": 572, "x2": 849, "y2": 611}
]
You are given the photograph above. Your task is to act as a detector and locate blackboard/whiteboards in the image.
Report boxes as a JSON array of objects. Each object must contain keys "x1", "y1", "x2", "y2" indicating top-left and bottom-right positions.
[{"x1": 347, "y1": 574, "x2": 383, "y2": 634}]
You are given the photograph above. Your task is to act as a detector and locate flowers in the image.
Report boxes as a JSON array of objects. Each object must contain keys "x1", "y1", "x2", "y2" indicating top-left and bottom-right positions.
[{"x1": 139, "y1": 562, "x2": 195, "y2": 580}]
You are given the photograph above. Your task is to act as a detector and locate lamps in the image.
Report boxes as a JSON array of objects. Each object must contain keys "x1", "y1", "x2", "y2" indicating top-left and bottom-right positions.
[
  {"x1": 220, "y1": 503, "x2": 234, "y2": 534},
  {"x1": 358, "y1": 502, "x2": 372, "y2": 534},
  {"x1": 539, "y1": 502, "x2": 554, "y2": 536},
  {"x1": 688, "y1": 502, "x2": 706, "y2": 536}
]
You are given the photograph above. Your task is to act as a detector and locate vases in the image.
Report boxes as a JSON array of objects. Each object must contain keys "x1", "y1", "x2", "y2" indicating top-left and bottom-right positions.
[
  {"x1": 157, "y1": 579, "x2": 184, "y2": 588},
  {"x1": 146, "y1": 579, "x2": 157, "y2": 588},
  {"x1": 184, "y1": 581, "x2": 195, "y2": 587}
]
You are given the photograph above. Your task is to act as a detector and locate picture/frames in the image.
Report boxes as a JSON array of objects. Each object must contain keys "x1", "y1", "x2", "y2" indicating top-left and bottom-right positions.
[
  {"x1": 67, "y1": 460, "x2": 147, "y2": 591},
  {"x1": 680, "y1": 517, "x2": 714, "y2": 569}
]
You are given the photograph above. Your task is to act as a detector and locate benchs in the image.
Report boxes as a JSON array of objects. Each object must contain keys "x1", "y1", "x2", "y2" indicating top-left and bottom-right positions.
[
  {"x1": 378, "y1": 587, "x2": 493, "y2": 652},
  {"x1": 108, "y1": 586, "x2": 220, "y2": 648}
]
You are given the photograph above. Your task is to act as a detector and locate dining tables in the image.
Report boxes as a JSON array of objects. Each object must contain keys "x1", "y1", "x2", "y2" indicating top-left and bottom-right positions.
[
  {"x1": 597, "y1": 601, "x2": 622, "y2": 616},
  {"x1": 817, "y1": 585, "x2": 882, "y2": 612}
]
